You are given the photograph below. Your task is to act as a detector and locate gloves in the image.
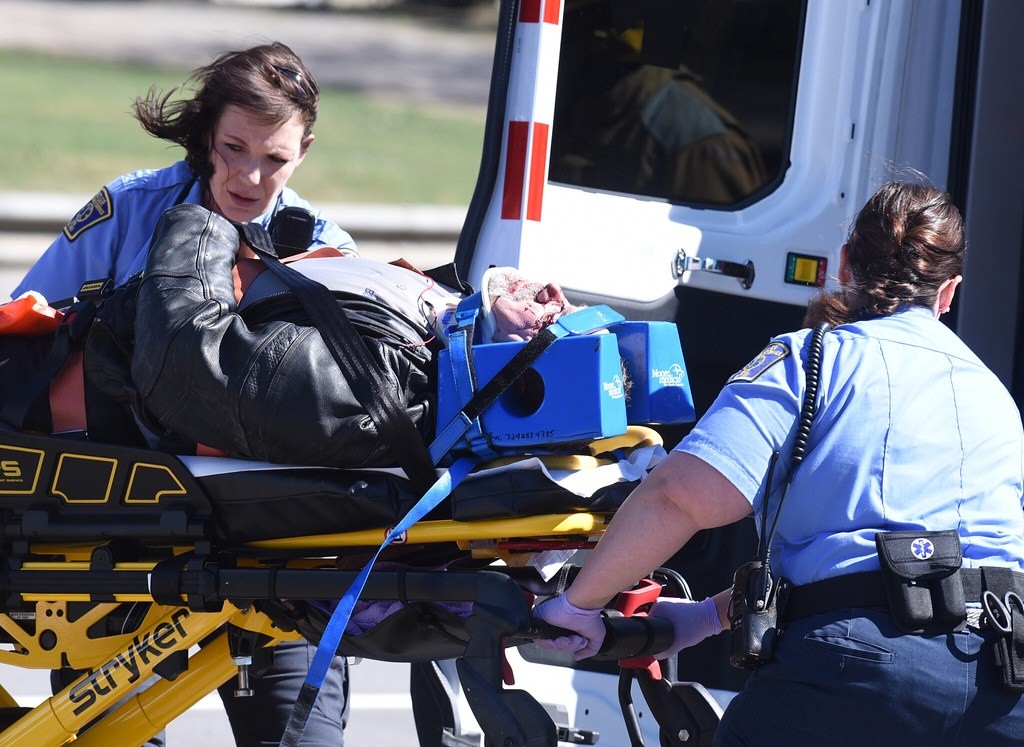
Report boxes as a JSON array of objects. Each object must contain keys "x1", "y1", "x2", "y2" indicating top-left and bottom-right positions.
[
  {"x1": 648, "y1": 596, "x2": 722, "y2": 660},
  {"x1": 532, "y1": 592, "x2": 606, "y2": 661}
]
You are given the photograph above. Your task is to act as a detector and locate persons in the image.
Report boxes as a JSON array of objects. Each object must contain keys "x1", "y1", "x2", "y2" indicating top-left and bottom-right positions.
[
  {"x1": 531, "y1": 180, "x2": 1024, "y2": 747},
  {"x1": 0, "y1": 203, "x2": 633, "y2": 463},
  {"x1": 9, "y1": 41, "x2": 362, "y2": 747},
  {"x1": 557, "y1": 23, "x2": 766, "y2": 204}
]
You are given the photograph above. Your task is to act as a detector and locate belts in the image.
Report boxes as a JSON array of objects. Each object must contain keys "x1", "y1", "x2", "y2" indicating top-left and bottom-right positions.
[{"x1": 863, "y1": 604, "x2": 1001, "y2": 629}]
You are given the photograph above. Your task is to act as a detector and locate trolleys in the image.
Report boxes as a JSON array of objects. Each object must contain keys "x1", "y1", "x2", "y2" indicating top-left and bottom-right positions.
[{"x1": 0, "y1": 420, "x2": 725, "y2": 747}]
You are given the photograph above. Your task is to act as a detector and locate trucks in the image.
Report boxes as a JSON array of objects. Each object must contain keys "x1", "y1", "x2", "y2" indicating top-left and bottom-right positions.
[{"x1": 452, "y1": 0, "x2": 1024, "y2": 424}]
